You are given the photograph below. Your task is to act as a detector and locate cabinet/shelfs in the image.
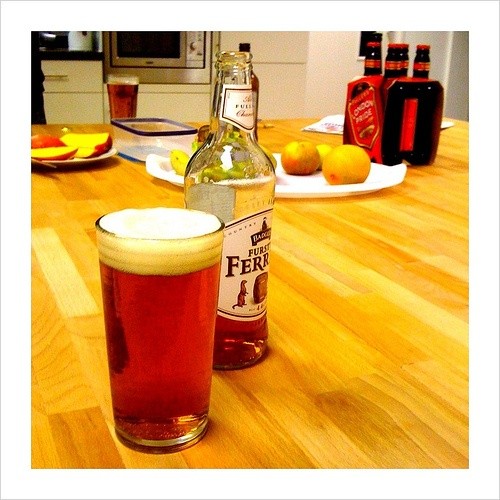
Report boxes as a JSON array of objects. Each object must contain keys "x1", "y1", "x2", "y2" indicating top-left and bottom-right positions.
[{"x1": 41, "y1": 54, "x2": 107, "y2": 124}]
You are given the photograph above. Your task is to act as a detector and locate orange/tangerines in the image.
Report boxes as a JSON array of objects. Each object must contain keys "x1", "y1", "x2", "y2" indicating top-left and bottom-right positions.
[{"x1": 281, "y1": 141, "x2": 371, "y2": 185}]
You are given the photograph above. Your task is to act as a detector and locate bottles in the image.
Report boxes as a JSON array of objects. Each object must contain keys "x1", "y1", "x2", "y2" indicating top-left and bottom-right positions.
[
  {"x1": 183, "y1": 51, "x2": 276, "y2": 370},
  {"x1": 238, "y1": 42, "x2": 259, "y2": 144},
  {"x1": 363, "y1": 40, "x2": 437, "y2": 167}
]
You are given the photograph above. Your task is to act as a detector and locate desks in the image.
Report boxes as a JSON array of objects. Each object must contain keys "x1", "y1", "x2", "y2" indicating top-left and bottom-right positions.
[{"x1": 31, "y1": 118, "x2": 470, "y2": 469}]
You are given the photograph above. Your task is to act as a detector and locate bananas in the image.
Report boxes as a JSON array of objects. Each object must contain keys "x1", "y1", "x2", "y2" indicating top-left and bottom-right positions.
[{"x1": 170, "y1": 150, "x2": 245, "y2": 183}]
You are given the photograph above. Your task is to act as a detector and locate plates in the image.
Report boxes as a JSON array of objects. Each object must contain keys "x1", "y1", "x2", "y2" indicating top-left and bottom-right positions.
[
  {"x1": 144, "y1": 153, "x2": 407, "y2": 198},
  {"x1": 31, "y1": 146, "x2": 117, "y2": 169}
]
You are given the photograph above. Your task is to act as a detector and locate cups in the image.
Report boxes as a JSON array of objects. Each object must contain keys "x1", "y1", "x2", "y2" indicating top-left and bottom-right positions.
[
  {"x1": 95, "y1": 207, "x2": 225, "y2": 455},
  {"x1": 107, "y1": 74, "x2": 140, "y2": 130}
]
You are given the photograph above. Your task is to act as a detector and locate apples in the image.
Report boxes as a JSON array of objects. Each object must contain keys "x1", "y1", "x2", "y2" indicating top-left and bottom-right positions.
[{"x1": 31, "y1": 133, "x2": 112, "y2": 161}]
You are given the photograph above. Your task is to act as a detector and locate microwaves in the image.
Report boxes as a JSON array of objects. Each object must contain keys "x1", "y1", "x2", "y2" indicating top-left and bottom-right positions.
[{"x1": 110, "y1": 31, "x2": 205, "y2": 69}]
